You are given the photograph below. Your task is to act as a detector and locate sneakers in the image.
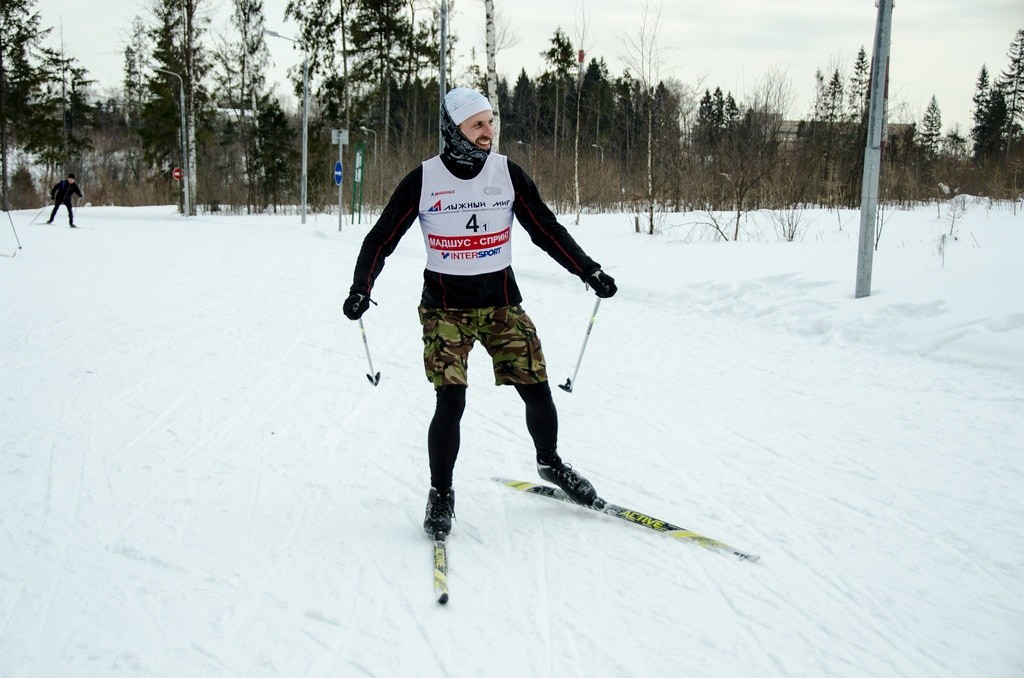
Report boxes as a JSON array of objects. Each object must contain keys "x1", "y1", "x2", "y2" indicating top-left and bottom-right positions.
[
  {"x1": 537, "y1": 456, "x2": 597, "y2": 506},
  {"x1": 423, "y1": 487, "x2": 456, "y2": 535}
]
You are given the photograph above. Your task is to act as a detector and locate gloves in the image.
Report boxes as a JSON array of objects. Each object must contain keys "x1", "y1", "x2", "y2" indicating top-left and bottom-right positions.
[
  {"x1": 51, "y1": 196, "x2": 55, "y2": 200},
  {"x1": 78, "y1": 193, "x2": 82, "y2": 197},
  {"x1": 586, "y1": 269, "x2": 617, "y2": 298},
  {"x1": 343, "y1": 293, "x2": 370, "y2": 320}
]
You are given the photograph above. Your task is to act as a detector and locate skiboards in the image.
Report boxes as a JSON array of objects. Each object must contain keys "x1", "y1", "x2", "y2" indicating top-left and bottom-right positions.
[
  {"x1": 33, "y1": 222, "x2": 94, "y2": 231},
  {"x1": 430, "y1": 474, "x2": 761, "y2": 607}
]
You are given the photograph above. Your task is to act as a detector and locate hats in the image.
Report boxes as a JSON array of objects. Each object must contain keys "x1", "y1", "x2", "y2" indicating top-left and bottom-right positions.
[
  {"x1": 444, "y1": 87, "x2": 493, "y2": 126},
  {"x1": 68, "y1": 173, "x2": 75, "y2": 179}
]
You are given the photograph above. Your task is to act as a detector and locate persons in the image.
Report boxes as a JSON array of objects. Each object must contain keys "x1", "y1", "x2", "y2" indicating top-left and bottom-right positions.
[
  {"x1": 344, "y1": 88, "x2": 618, "y2": 534},
  {"x1": 47, "y1": 173, "x2": 83, "y2": 227}
]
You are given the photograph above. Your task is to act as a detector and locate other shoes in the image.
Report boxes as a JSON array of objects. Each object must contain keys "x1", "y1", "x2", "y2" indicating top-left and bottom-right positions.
[
  {"x1": 70, "y1": 225, "x2": 76, "y2": 227},
  {"x1": 47, "y1": 220, "x2": 53, "y2": 223}
]
